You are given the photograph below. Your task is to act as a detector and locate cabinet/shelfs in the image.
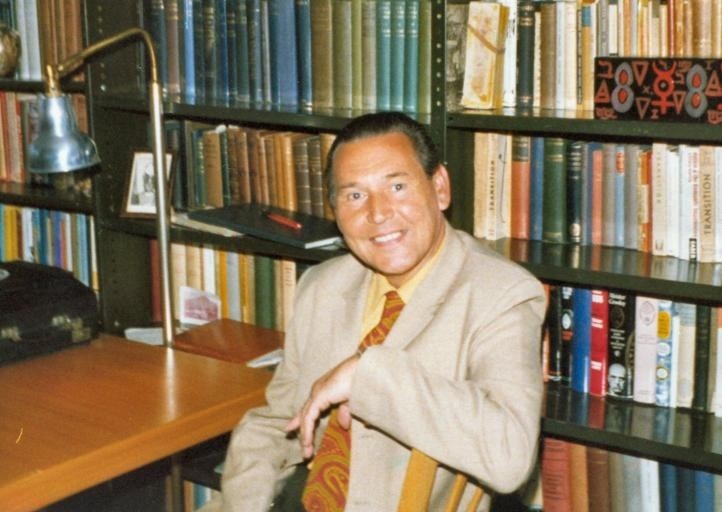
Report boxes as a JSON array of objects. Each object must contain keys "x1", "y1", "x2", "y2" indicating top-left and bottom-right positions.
[{"x1": 1, "y1": 0, "x2": 722, "y2": 474}]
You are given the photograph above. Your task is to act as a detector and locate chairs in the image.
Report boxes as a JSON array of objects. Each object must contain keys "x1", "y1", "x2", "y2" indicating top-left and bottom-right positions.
[{"x1": 396, "y1": 447, "x2": 485, "y2": 512}]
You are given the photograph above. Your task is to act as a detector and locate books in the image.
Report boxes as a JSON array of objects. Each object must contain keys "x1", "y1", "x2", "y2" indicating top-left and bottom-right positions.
[
  {"x1": 183, "y1": 480, "x2": 219, "y2": 510},
  {"x1": 540, "y1": 284, "x2": 722, "y2": 418},
  {"x1": 147, "y1": 120, "x2": 343, "y2": 250},
  {"x1": 132, "y1": 0, "x2": 431, "y2": 114},
  {"x1": 1, "y1": 1, "x2": 98, "y2": 295},
  {"x1": 461, "y1": 0, "x2": 721, "y2": 122},
  {"x1": 515, "y1": 437, "x2": 722, "y2": 511},
  {"x1": 149, "y1": 238, "x2": 309, "y2": 368}
]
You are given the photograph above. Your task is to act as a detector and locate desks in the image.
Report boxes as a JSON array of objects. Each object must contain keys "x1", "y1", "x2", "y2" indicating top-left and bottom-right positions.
[{"x1": 2, "y1": 335, "x2": 280, "y2": 512}]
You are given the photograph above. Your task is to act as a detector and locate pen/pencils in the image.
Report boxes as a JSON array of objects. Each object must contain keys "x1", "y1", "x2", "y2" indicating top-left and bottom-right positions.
[{"x1": 261, "y1": 207, "x2": 302, "y2": 230}]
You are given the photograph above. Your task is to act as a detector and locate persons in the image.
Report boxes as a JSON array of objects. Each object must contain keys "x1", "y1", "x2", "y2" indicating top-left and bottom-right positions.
[{"x1": 218, "y1": 112, "x2": 547, "y2": 511}]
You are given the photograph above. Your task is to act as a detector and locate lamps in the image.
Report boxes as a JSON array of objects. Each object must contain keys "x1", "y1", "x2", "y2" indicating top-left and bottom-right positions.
[{"x1": 25, "y1": 29, "x2": 176, "y2": 347}]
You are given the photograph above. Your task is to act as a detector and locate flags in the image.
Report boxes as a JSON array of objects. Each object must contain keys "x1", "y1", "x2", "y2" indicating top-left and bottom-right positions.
[{"x1": 472, "y1": 131, "x2": 722, "y2": 265}]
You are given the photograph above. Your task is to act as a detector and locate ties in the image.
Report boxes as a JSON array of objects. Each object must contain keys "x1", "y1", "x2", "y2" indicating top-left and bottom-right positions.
[{"x1": 302, "y1": 291, "x2": 405, "y2": 512}]
